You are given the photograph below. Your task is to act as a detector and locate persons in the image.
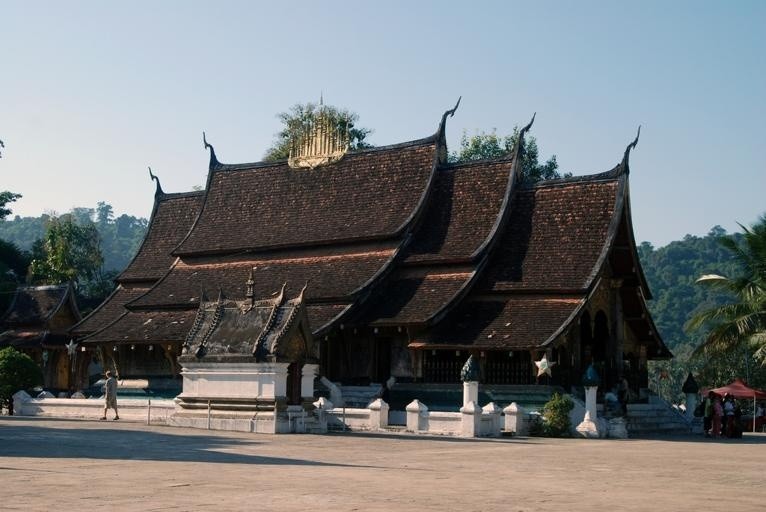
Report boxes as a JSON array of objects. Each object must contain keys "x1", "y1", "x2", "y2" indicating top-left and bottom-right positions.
[
  {"x1": 99, "y1": 369, "x2": 120, "y2": 420},
  {"x1": 27, "y1": 384, "x2": 100, "y2": 399},
  {"x1": 671, "y1": 390, "x2": 766, "y2": 441},
  {"x1": 566, "y1": 372, "x2": 647, "y2": 424}
]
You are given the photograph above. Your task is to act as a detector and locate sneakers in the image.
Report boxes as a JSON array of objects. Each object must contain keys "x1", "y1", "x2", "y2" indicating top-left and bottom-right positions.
[{"x1": 100, "y1": 415, "x2": 119, "y2": 420}]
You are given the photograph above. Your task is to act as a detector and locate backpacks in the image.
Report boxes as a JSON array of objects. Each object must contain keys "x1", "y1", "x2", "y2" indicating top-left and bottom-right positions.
[{"x1": 694, "y1": 400, "x2": 706, "y2": 417}]
